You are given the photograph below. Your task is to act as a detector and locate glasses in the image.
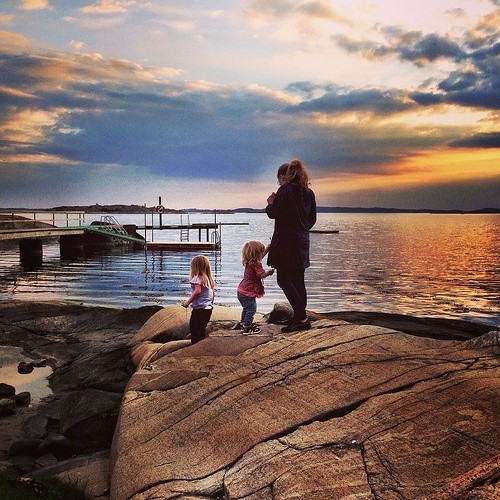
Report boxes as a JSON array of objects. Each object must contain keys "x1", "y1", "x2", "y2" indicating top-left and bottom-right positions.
[{"x1": 277, "y1": 176, "x2": 284, "y2": 185}]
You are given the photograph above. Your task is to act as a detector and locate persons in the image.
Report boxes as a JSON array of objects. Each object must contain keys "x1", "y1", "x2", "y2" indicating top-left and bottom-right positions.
[
  {"x1": 266, "y1": 160, "x2": 317, "y2": 333},
  {"x1": 181, "y1": 256, "x2": 215, "y2": 345},
  {"x1": 237, "y1": 240, "x2": 275, "y2": 335}
]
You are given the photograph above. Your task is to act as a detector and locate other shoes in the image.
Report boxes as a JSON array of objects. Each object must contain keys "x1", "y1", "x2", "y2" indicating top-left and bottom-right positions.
[
  {"x1": 281, "y1": 319, "x2": 311, "y2": 332},
  {"x1": 242, "y1": 326, "x2": 261, "y2": 335},
  {"x1": 278, "y1": 316, "x2": 294, "y2": 325}
]
